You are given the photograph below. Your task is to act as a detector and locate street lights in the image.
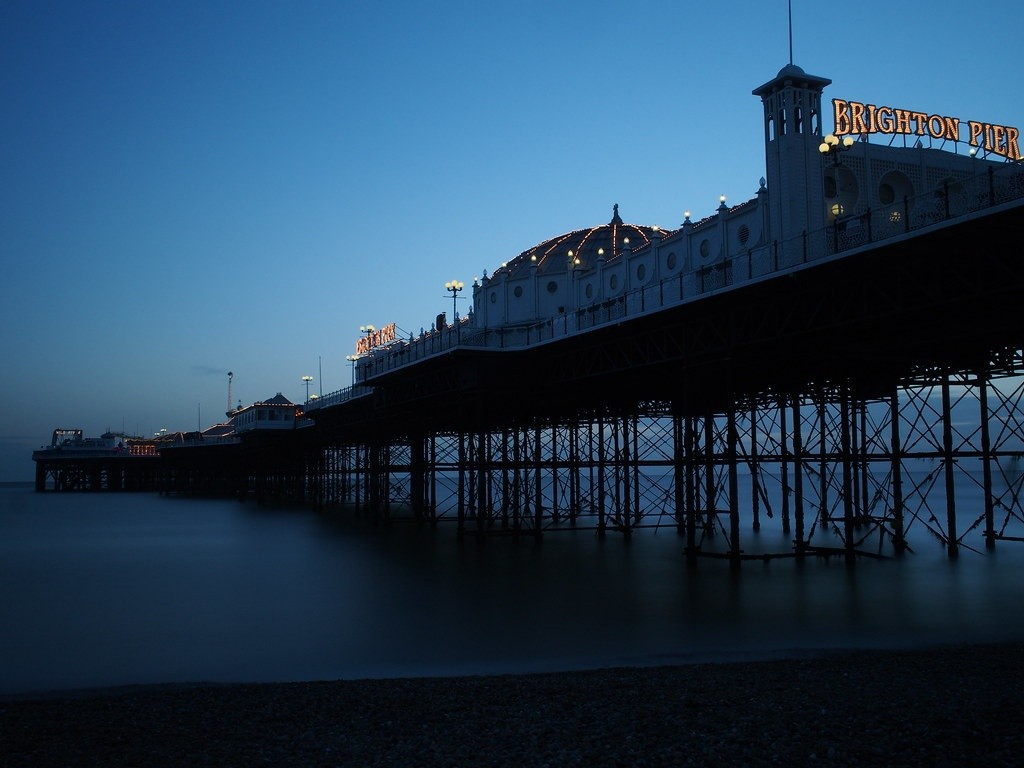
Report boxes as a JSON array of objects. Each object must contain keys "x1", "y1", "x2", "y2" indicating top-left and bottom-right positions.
[
  {"x1": 309, "y1": 395, "x2": 318, "y2": 411},
  {"x1": 819, "y1": 134, "x2": 856, "y2": 255},
  {"x1": 153, "y1": 428, "x2": 167, "y2": 438},
  {"x1": 301, "y1": 376, "x2": 314, "y2": 410},
  {"x1": 361, "y1": 325, "x2": 376, "y2": 378},
  {"x1": 443, "y1": 280, "x2": 467, "y2": 328}
]
